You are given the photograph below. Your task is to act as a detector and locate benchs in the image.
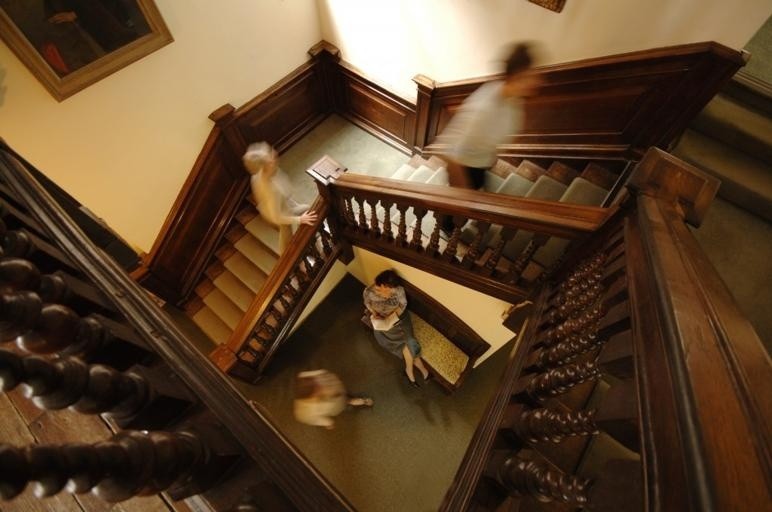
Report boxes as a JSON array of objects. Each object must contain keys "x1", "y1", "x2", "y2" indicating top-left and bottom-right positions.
[{"x1": 361, "y1": 277, "x2": 491, "y2": 393}]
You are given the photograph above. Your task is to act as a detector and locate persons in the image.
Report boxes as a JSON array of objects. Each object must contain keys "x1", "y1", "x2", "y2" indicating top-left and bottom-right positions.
[
  {"x1": 240, "y1": 138, "x2": 320, "y2": 258},
  {"x1": 362, "y1": 266, "x2": 431, "y2": 390},
  {"x1": 289, "y1": 366, "x2": 377, "y2": 432},
  {"x1": 427, "y1": 38, "x2": 549, "y2": 251}
]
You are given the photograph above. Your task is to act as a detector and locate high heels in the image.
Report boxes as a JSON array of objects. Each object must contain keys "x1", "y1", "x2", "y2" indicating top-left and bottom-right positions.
[{"x1": 403, "y1": 369, "x2": 432, "y2": 389}]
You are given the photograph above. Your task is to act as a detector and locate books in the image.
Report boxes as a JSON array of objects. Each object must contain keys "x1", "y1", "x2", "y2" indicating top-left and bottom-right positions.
[{"x1": 370, "y1": 311, "x2": 402, "y2": 331}]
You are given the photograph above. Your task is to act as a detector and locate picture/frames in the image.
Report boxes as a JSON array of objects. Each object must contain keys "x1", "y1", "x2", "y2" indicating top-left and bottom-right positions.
[{"x1": 0, "y1": 0, "x2": 175, "y2": 103}]
